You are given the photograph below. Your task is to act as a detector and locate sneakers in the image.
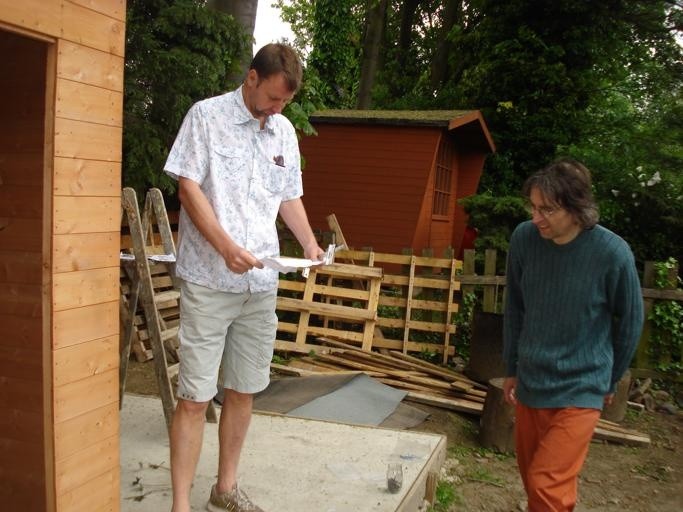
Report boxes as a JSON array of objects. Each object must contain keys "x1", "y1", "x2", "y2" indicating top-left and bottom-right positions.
[{"x1": 207, "y1": 483, "x2": 266, "y2": 512}]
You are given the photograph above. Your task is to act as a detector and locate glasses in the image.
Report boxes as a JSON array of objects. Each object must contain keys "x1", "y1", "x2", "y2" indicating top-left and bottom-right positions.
[{"x1": 522, "y1": 199, "x2": 564, "y2": 216}]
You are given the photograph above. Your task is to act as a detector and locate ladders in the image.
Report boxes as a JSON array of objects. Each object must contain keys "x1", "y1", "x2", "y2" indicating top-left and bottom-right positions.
[{"x1": 119, "y1": 187, "x2": 218, "y2": 439}]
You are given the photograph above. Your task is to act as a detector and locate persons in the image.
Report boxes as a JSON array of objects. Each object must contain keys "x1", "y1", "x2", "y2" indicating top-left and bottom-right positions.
[
  {"x1": 162, "y1": 43, "x2": 328, "y2": 511},
  {"x1": 500, "y1": 158, "x2": 646, "y2": 511}
]
